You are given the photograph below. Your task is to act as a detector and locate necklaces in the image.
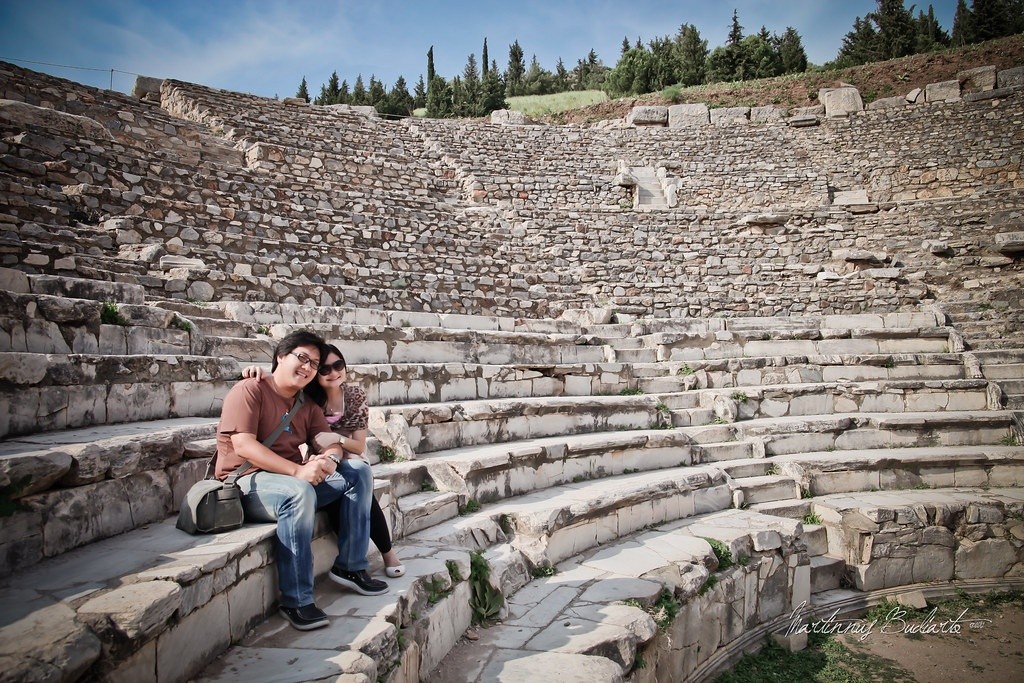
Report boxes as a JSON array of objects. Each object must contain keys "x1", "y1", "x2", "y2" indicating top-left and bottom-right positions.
[{"x1": 330, "y1": 409, "x2": 333, "y2": 411}]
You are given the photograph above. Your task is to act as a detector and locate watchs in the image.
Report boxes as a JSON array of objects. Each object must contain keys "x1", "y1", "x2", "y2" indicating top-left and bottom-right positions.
[
  {"x1": 327, "y1": 454, "x2": 342, "y2": 470},
  {"x1": 338, "y1": 435, "x2": 346, "y2": 445}
]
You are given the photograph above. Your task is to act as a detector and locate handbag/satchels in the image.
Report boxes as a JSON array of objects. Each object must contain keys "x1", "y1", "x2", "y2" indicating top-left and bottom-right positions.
[{"x1": 176, "y1": 480, "x2": 244, "y2": 535}]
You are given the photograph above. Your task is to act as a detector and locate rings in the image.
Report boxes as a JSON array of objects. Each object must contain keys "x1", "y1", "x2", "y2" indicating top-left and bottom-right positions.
[{"x1": 317, "y1": 478, "x2": 321, "y2": 481}]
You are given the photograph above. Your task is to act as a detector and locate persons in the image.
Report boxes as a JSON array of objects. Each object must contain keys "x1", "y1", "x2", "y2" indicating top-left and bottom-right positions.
[
  {"x1": 214, "y1": 328, "x2": 389, "y2": 630},
  {"x1": 242, "y1": 343, "x2": 406, "y2": 578}
]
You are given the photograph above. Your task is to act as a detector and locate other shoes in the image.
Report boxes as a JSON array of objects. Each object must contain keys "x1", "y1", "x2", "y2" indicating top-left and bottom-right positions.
[
  {"x1": 279, "y1": 603, "x2": 330, "y2": 631},
  {"x1": 329, "y1": 561, "x2": 390, "y2": 596},
  {"x1": 386, "y1": 565, "x2": 405, "y2": 577}
]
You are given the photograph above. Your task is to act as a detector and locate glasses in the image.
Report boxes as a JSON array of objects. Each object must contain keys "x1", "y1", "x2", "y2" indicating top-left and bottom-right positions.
[
  {"x1": 290, "y1": 351, "x2": 321, "y2": 370},
  {"x1": 318, "y1": 359, "x2": 345, "y2": 376}
]
